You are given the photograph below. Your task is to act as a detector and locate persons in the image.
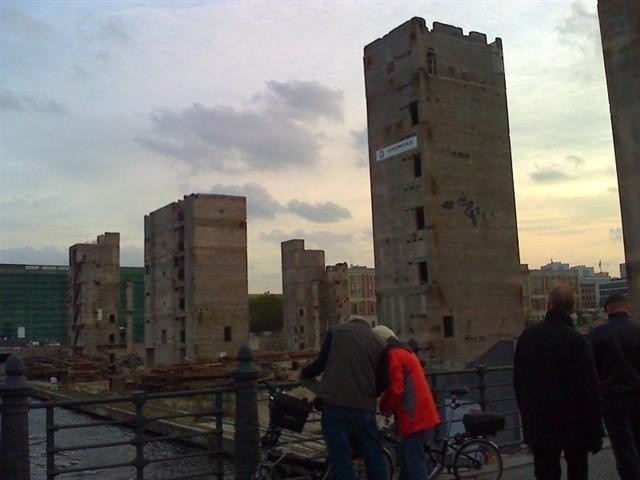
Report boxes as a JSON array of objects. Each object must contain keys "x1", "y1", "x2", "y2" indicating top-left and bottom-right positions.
[
  {"x1": 298, "y1": 313, "x2": 387, "y2": 479},
  {"x1": 373, "y1": 325, "x2": 442, "y2": 479},
  {"x1": 587, "y1": 291, "x2": 640, "y2": 480},
  {"x1": 512, "y1": 284, "x2": 606, "y2": 479}
]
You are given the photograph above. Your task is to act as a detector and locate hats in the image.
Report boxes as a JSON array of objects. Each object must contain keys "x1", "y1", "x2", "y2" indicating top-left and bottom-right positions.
[
  {"x1": 347, "y1": 314, "x2": 371, "y2": 328},
  {"x1": 602, "y1": 293, "x2": 627, "y2": 313}
]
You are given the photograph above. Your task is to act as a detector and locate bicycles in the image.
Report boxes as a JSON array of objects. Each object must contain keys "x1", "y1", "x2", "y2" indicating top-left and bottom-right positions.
[
  {"x1": 359, "y1": 387, "x2": 506, "y2": 480},
  {"x1": 256, "y1": 379, "x2": 394, "y2": 479}
]
